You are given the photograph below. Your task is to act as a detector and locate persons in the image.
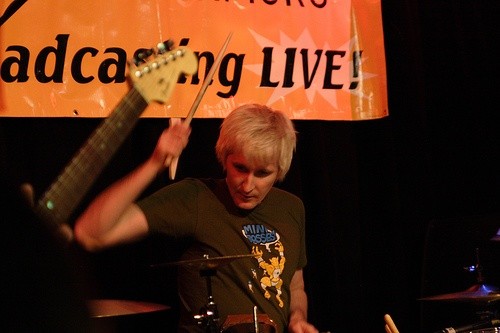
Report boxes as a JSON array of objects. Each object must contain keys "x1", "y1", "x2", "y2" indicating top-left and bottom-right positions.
[
  {"x1": 385, "y1": 314, "x2": 400, "y2": 333},
  {"x1": 74, "y1": 105, "x2": 318, "y2": 333},
  {"x1": 0, "y1": 149, "x2": 73, "y2": 333}
]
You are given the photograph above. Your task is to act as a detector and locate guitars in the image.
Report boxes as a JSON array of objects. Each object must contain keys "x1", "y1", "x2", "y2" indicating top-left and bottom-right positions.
[{"x1": 33, "y1": 42, "x2": 197, "y2": 230}]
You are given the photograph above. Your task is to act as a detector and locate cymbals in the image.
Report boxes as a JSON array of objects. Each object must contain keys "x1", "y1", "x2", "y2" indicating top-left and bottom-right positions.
[
  {"x1": 172, "y1": 252, "x2": 262, "y2": 265},
  {"x1": 90, "y1": 297, "x2": 167, "y2": 317},
  {"x1": 422, "y1": 284, "x2": 500, "y2": 301}
]
